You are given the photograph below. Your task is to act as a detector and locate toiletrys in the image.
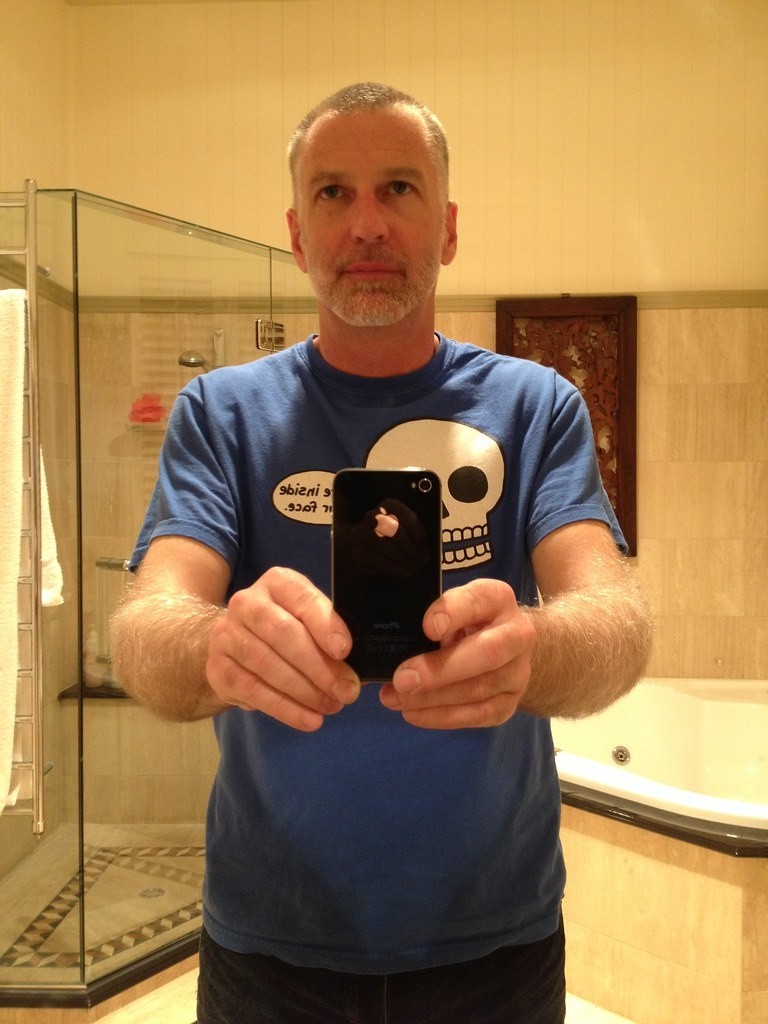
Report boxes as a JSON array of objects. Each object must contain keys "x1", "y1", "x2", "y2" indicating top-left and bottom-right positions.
[{"x1": 84, "y1": 624, "x2": 102, "y2": 687}]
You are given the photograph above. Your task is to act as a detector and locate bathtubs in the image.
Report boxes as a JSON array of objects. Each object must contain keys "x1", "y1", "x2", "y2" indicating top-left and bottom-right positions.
[{"x1": 550, "y1": 680, "x2": 768, "y2": 1024}]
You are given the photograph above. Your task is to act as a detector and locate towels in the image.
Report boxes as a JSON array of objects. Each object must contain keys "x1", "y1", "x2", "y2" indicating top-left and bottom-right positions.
[{"x1": 0, "y1": 287, "x2": 65, "y2": 807}]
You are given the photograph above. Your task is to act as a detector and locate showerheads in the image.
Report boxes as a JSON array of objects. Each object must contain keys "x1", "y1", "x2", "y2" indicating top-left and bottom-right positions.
[{"x1": 179, "y1": 351, "x2": 211, "y2": 373}]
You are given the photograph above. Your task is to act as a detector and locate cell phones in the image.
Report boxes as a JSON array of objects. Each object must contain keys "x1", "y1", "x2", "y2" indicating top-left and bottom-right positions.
[{"x1": 328, "y1": 465, "x2": 444, "y2": 685}]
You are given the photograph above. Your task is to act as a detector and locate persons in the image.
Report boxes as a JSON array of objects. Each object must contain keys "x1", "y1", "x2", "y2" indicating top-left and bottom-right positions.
[{"x1": 109, "y1": 81, "x2": 654, "y2": 1024}]
